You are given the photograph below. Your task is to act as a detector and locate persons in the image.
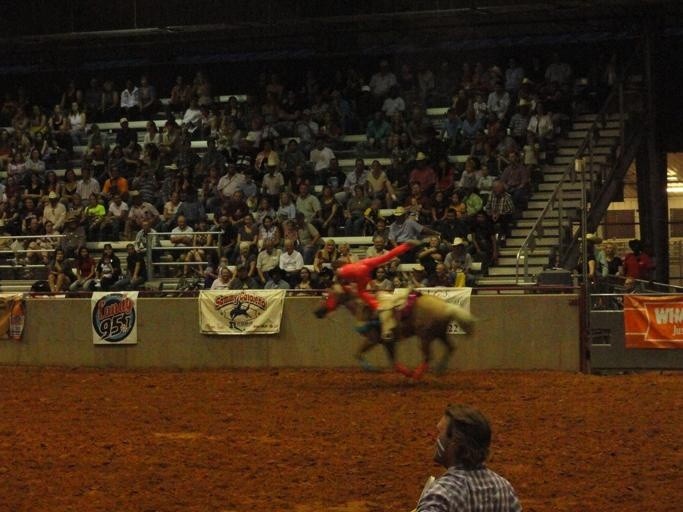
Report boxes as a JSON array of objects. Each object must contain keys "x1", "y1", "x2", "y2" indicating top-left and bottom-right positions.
[
  {"x1": 416, "y1": 403, "x2": 523, "y2": 512},
  {"x1": 0, "y1": 53, "x2": 615, "y2": 294},
  {"x1": 576, "y1": 233, "x2": 654, "y2": 307}
]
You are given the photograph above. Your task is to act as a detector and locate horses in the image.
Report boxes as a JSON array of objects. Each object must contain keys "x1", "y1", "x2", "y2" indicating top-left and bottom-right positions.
[{"x1": 312, "y1": 281, "x2": 479, "y2": 387}]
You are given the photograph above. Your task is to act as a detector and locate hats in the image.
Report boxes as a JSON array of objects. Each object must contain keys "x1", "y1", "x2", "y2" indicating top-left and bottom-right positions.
[
  {"x1": 119, "y1": 116, "x2": 128, "y2": 125},
  {"x1": 393, "y1": 206, "x2": 405, "y2": 216},
  {"x1": 452, "y1": 237, "x2": 465, "y2": 246},
  {"x1": 415, "y1": 151, "x2": 428, "y2": 161},
  {"x1": 47, "y1": 190, "x2": 58, "y2": 199}
]
